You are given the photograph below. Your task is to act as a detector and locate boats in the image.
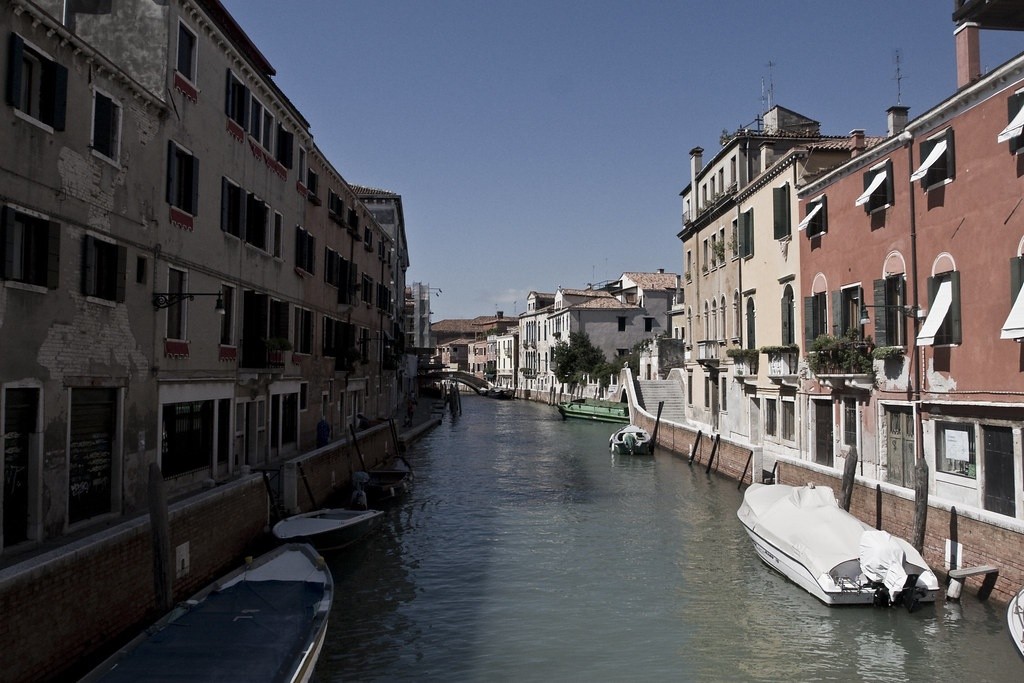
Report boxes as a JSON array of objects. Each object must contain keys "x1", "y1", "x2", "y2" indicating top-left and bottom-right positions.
[
  {"x1": 558, "y1": 398, "x2": 630, "y2": 424},
  {"x1": 608, "y1": 422, "x2": 651, "y2": 456},
  {"x1": 354, "y1": 455, "x2": 413, "y2": 501},
  {"x1": 488, "y1": 390, "x2": 514, "y2": 400},
  {"x1": 78, "y1": 536, "x2": 335, "y2": 683},
  {"x1": 1006, "y1": 587, "x2": 1024, "y2": 659},
  {"x1": 738, "y1": 478, "x2": 940, "y2": 609},
  {"x1": 272, "y1": 504, "x2": 384, "y2": 550}
]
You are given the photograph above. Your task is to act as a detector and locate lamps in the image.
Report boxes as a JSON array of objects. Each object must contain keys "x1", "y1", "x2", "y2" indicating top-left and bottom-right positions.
[
  {"x1": 421, "y1": 287, "x2": 442, "y2": 296},
  {"x1": 152, "y1": 290, "x2": 225, "y2": 314},
  {"x1": 356, "y1": 338, "x2": 390, "y2": 348},
  {"x1": 861, "y1": 304, "x2": 921, "y2": 324}
]
